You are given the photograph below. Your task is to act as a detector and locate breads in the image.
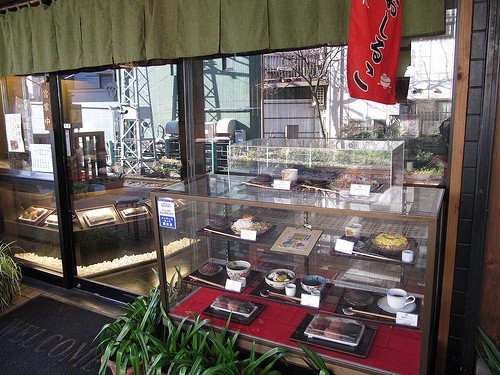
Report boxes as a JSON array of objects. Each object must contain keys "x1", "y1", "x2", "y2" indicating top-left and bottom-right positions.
[
  {"x1": 308, "y1": 318, "x2": 362, "y2": 343},
  {"x1": 216, "y1": 296, "x2": 254, "y2": 314}
]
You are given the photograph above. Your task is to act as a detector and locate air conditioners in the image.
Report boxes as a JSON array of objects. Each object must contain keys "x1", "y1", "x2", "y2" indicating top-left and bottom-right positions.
[{"x1": 312, "y1": 86, "x2": 324, "y2": 107}]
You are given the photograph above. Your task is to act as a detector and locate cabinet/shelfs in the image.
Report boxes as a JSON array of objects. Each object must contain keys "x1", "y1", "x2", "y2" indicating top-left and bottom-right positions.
[
  {"x1": 149, "y1": 173, "x2": 447, "y2": 375},
  {"x1": 0, "y1": 175, "x2": 65, "y2": 280},
  {"x1": 68, "y1": 180, "x2": 204, "y2": 280}
]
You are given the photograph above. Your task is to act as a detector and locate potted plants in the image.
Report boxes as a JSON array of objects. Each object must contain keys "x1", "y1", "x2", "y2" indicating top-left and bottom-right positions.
[{"x1": 93, "y1": 286, "x2": 168, "y2": 375}]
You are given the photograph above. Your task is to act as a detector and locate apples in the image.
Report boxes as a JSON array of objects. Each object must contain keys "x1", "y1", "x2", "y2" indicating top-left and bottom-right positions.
[{"x1": 22, "y1": 207, "x2": 45, "y2": 220}]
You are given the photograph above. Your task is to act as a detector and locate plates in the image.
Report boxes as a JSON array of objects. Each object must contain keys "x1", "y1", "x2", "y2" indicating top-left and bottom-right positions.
[
  {"x1": 44, "y1": 213, "x2": 75, "y2": 226},
  {"x1": 377, "y1": 296, "x2": 415, "y2": 313},
  {"x1": 211, "y1": 298, "x2": 258, "y2": 317},
  {"x1": 231, "y1": 222, "x2": 270, "y2": 234},
  {"x1": 304, "y1": 314, "x2": 365, "y2": 346},
  {"x1": 177, "y1": 199, "x2": 196, "y2": 205},
  {"x1": 83, "y1": 212, "x2": 116, "y2": 226},
  {"x1": 145, "y1": 197, "x2": 179, "y2": 211},
  {"x1": 18, "y1": 208, "x2": 47, "y2": 222},
  {"x1": 121, "y1": 206, "x2": 148, "y2": 218},
  {"x1": 369, "y1": 232, "x2": 411, "y2": 251},
  {"x1": 198, "y1": 266, "x2": 222, "y2": 276},
  {"x1": 344, "y1": 291, "x2": 374, "y2": 305}
]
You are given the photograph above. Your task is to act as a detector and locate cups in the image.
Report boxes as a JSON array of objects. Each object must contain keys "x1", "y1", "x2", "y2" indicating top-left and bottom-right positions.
[
  {"x1": 285, "y1": 283, "x2": 296, "y2": 296},
  {"x1": 387, "y1": 288, "x2": 415, "y2": 309},
  {"x1": 345, "y1": 224, "x2": 362, "y2": 238},
  {"x1": 401, "y1": 250, "x2": 413, "y2": 262},
  {"x1": 311, "y1": 290, "x2": 320, "y2": 302},
  {"x1": 282, "y1": 169, "x2": 298, "y2": 187}
]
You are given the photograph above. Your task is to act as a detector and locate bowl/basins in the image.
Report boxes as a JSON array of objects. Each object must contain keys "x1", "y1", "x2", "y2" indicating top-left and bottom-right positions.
[
  {"x1": 265, "y1": 269, "x2": 296, "y2": 289},
  {"x1": 226, "y1": 260, "x2": 251, "y2": 279},
  {"x1": 301, "y1": 274, "x2": 325, "y2": 293},
  {"x1": 209, "y1": 221, "x2": 232, "y2": 230}
]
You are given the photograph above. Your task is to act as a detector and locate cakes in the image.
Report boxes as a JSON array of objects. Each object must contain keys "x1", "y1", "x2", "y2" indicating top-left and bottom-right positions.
[
  {"x1": 123, "y1": 206, "x2": 147, "y2": 216},
  {"x1": 85, "y1": 207, "x2": 114, "y2": 224},
  {"x1": 231, "y1": 215, "x2": 270, "y2": 234},
  {"x1": 46, "y1": 215, "x2": 58, "y2": 223},
  {"x1": 372, "y1": 231, "x2": 410, "y2": 251},
  {"x1": 335, "y1": 173, "x2": 379, "y2": 191}
]
